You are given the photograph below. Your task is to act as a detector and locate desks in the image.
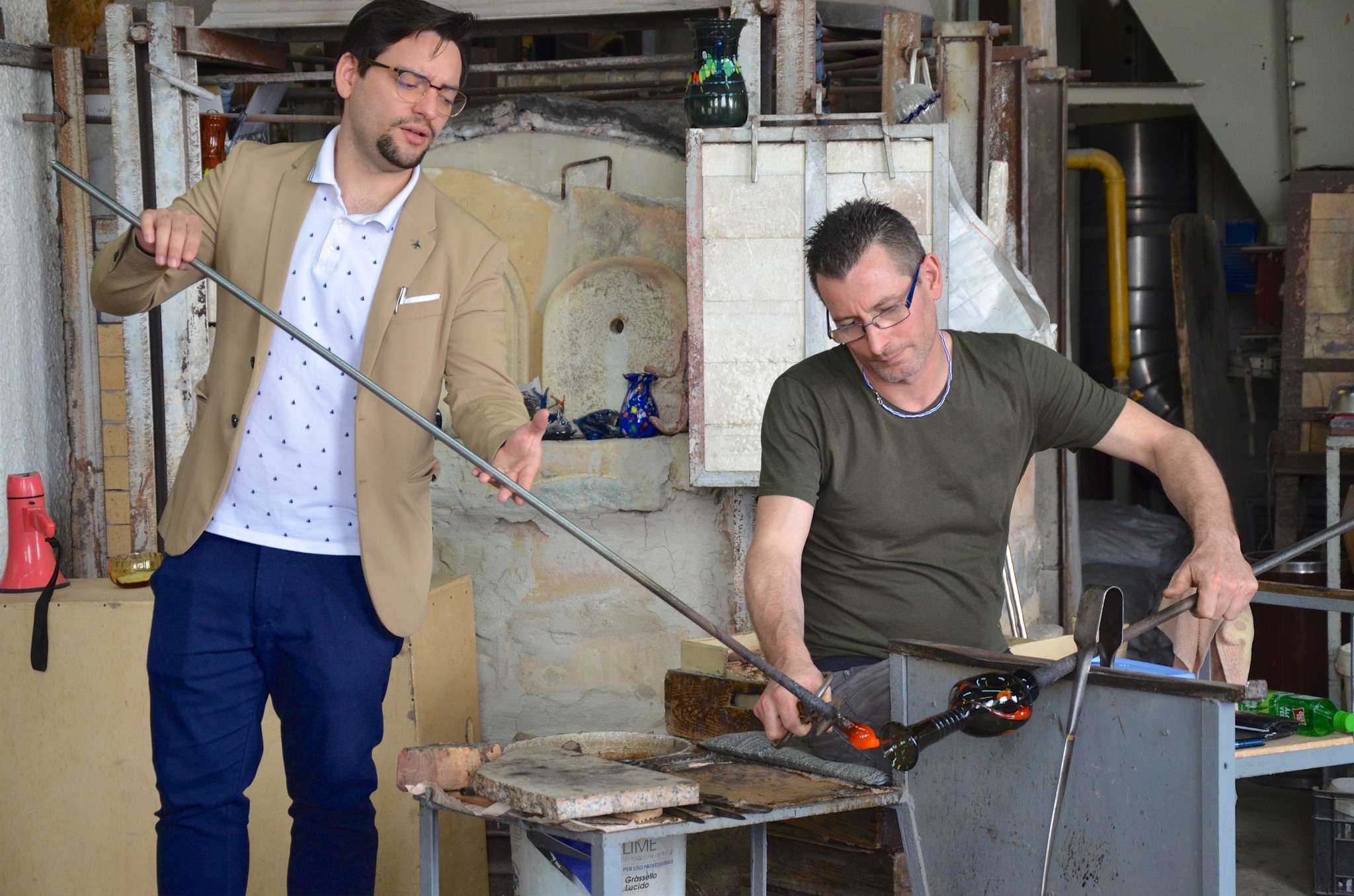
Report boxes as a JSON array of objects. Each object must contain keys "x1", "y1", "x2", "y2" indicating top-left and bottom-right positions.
[
  {"x1": 1044, "y1": 657, "x2": 1353, "y2": 811},
  {"x1": 412, "y1": 738, "x2": 941, "y2": 896}
]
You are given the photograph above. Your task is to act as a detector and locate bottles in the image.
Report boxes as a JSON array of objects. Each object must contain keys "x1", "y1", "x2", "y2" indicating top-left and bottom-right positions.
[
  {"x1": 619, "y1": 373, "x2": 659, "y2": 439},
  {"x1": 684, "y1": 18, "x2": 749, "y2": 128},
  {"x1": 199, "y1": 114, "x2": 230, "y2": 179},
  {"x1": 893, "y1": 78, "x2": 942, "y2": 124},
  {"x1": 1238, "y1": 689, "x2": 1354, "y2": 737}
]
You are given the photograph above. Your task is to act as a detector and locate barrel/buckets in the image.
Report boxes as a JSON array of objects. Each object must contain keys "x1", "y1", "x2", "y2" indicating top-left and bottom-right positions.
[
  {"x1": 1328, "y1": 777, "x2": 1354, "y2": 892},
  {"x1": 502, "y1": 731, "x2": 692, "y2": 896},
  {"x1": 1337, "y1": 643, "x2": 1352, "y2": 713}
]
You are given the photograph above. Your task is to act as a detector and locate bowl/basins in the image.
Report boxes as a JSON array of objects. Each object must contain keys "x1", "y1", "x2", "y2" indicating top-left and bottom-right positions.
[{"x1": 108, "y1": 552, "x2": 163, "y2": 589}]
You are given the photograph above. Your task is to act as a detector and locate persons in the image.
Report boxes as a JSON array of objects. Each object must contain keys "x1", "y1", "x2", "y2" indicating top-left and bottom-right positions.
[
  {"x1": 743, "y1": 198, "x2": 1258, "y2": 768},
  {"x1": 87, "y1": 0, "x2": 550, "y2": 896}
]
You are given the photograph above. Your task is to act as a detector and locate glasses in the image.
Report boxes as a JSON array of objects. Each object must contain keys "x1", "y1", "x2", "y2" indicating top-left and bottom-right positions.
[
  {"x1": 825, "y1": 255, "x2": 925, "y2": 346},
  {"x1": 353, "y1": 55, "x2": 470, "y2": 118}
]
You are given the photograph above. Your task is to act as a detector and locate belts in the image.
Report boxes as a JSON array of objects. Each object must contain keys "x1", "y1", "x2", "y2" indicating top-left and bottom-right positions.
[{"x1": 812, "y1": 654, "x2": 884, "y2": 672}]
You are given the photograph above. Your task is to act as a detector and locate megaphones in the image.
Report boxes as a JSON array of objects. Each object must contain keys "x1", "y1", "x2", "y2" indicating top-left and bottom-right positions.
[{"x1": 0, "y1": 471, "x2": 70, "y2": 593}]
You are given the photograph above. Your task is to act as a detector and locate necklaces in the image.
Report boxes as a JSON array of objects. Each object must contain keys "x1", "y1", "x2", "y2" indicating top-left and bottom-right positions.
[{"x1": 861, "y1": 330, "x2": 952, "y2": 418}]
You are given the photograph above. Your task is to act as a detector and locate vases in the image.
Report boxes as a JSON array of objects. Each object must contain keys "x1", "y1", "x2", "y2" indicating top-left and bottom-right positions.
[
  {"x1": 616, "y1": 365, "x2": 661, "y2": 440},
  {"x1": 890, "y1": 71, "x2": 949, "y2": 128},
  {"x1": 675, "y1": 12, "x2": 754, "y2": 130}
]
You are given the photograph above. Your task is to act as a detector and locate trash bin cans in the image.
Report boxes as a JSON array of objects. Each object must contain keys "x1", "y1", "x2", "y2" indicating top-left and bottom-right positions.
[{"x1": 1244, "y1": 551, "x2": 1352, "y2": 699}]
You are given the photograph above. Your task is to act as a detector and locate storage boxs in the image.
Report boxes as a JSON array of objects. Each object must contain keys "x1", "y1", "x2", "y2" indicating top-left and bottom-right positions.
[{"x1": 1307, "y1": 785, "x2": 1354, "y2": 896}]
